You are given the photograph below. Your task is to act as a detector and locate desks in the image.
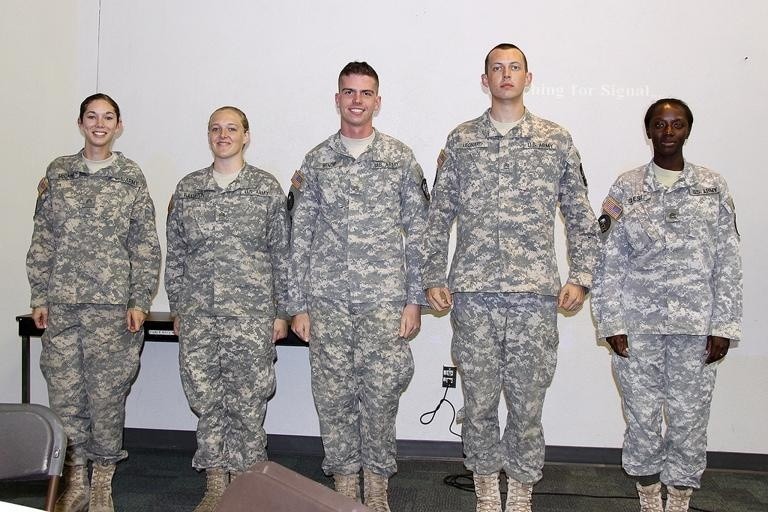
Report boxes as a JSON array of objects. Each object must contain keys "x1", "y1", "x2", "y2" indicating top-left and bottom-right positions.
[{"x1": 16, "y1": 312, "x2": 309, "y2": 405}]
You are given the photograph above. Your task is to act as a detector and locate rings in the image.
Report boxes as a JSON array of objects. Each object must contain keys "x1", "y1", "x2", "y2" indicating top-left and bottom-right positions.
[{"x1": 720, "y1": 353, "x2": 724, "y2": 356}]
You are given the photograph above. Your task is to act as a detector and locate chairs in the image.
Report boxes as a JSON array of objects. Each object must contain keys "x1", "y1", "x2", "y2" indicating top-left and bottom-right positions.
[
  {"x1": 214, "y1": 462, "x2": 377, "y2": 511},
  {"x1": 0, "y1": 404, "x2": 67, "y2": 512}
]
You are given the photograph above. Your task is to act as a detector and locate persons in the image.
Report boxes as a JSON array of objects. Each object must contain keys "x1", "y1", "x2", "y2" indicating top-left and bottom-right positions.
[
  {"x1": 25, "y1": 94, "x2": 161, "y2": 512},
  {"x1": 164, "y1": 107, "x2": 287, "y2": 512},
  {"x1": 424, "y1": 44, "x2": 602, "y2": 512},
  {"x1": 590, "y1": 99, "x2": 743, "y2": 512},
  {"x1": 288, "y1": 63, "x2": 431, "y2": 512}
]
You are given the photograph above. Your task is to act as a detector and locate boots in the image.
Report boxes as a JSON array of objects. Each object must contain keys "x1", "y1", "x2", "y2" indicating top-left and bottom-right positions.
[{"x1": 54, "y1": 463, "x2": 231, "y2": 512}]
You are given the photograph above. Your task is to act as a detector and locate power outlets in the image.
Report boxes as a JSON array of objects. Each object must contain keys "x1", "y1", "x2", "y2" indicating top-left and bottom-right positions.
[{"x1": 442, "y1": 366, "x2": 457, "y2": 388}]
